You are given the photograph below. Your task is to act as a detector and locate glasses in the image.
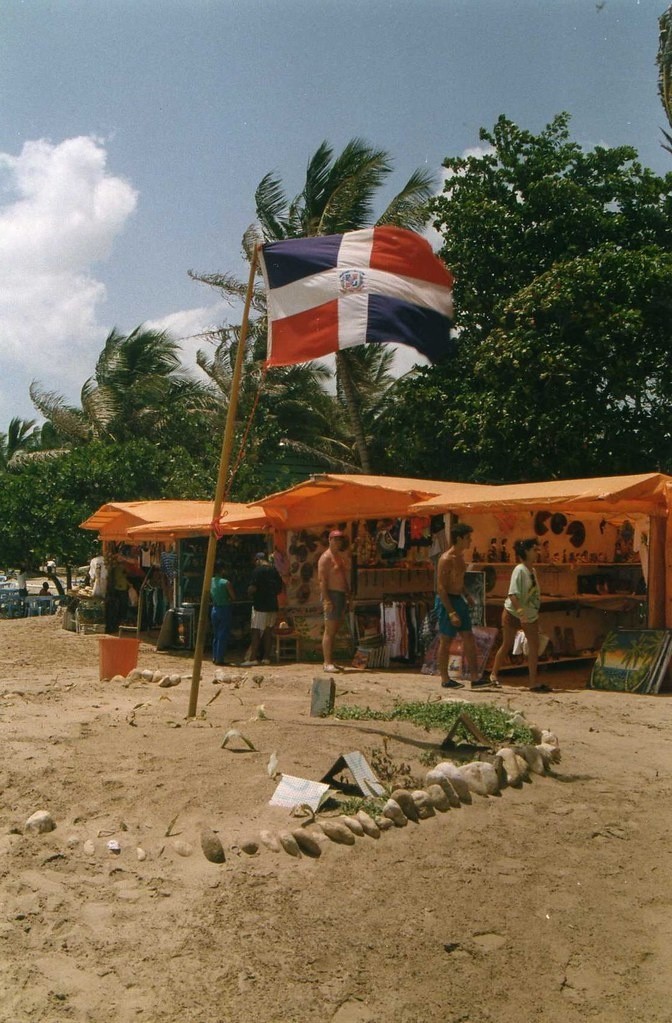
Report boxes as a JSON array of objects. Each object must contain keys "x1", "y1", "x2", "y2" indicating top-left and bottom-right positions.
[{"x1": 334, "y1": 538, "x2": 343, "y2": 541}]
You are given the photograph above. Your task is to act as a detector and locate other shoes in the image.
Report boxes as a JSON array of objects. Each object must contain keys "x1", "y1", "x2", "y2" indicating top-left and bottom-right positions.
[
  {"x1": 212, "y1": 660, "x2": 230, "y2": 666},
  {"x1": 322, "y1": 663, "x2": 344, "y2": 673},
  {"x1": 240, "y1": 660, "x2": 258, "y2": 666},
  {"x1": 261, "y1": 659, "x2": 270, "y2": 664}
]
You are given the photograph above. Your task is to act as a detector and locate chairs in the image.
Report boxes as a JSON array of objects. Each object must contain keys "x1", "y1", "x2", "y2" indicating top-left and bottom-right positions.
[{"x1": 271, "y1": 612, "x2": 301, "y2": 662}]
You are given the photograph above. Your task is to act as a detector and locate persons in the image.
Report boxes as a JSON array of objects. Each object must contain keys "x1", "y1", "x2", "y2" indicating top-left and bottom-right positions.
[
  {"x1": 433, "y1": 524, "x2": 493, "y2": 689},
  {"x1": 243, "y1": 554, "x2": 282, "y2": 662},
  {"x1": 17, "y1": 569, "x2": 28, "y2": 596},
  {"x1": 318, "y1": 530, "x2": 348, "y2": 673},
  {"x1": 46, "y1": 558, "x2": 56, "y2": 580},
  {"x1": 40, "y1": 582, "x2": 51, "y2": 596},
  {"x1": 210, "y1": 560, "x2": 236, "y2": 665},
  {"x1": 489, "y1": 538, "x2": 550, "y2": 692}
]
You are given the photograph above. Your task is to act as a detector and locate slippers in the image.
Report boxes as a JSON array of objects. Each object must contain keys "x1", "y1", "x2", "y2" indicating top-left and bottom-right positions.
[
  {"x1": 491, "y1": 680, "x2": 502, "y2": 688},
  {"x1": 529, "y1": 683, "x2": 553, "y2": 691}
]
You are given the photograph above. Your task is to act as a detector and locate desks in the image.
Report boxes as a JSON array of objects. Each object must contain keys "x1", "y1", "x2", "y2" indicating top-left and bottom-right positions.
[
  {"x1": 24, "y1": 595, "x2": 65, "y2": 615},
  {"x1": 349, "y1": 597, "x2": 435, "y2": 664},
  {"x1": 182, "y1": 600, "x2": 253, "y2": 649}
]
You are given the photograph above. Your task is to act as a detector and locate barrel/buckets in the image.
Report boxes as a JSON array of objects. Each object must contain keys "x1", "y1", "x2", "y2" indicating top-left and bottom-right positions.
[{"x1": 98, "y1": 638, "x2": 140, "y2": 681}]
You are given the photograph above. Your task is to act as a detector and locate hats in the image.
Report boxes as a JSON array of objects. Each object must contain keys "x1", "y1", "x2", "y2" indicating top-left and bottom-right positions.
[
  {"x1": 329, "y1": 530, "x2": 345, "y2": 538},
  {"x1": 254, "y1": 552, "x2": 266, "y2": 560}
]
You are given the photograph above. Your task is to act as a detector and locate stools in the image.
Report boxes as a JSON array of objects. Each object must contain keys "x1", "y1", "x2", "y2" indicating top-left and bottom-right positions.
[{"x1": 119, "y1": 625, "x2": 138, "y2": 638}]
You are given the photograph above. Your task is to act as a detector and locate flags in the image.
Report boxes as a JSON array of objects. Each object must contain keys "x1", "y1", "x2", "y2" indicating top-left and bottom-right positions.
[{"x1": 258, "y1": 226, "x2": 453, "y2": 366}]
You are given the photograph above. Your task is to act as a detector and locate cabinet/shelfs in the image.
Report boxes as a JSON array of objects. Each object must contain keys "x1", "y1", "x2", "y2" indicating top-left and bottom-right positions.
[{"x1": 462, "y1": 563, "x2": 643, "y2": 675}]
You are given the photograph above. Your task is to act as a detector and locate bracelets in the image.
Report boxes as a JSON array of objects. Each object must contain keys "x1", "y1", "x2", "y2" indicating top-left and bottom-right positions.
[
  {"x1": 465, "y1": 594, "x2": 468, "y2": 598},
  {"x1": 517, "y1": 609, "x2": 523, "y2": 616},
  {"x1": 449, "y1": 612, "x2": 456, "y2": 617}
]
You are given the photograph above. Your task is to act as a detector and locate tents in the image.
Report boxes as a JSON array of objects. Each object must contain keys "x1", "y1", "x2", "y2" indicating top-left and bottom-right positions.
[{"x1": 77, "y1": 474, "x2": 672, "y2": 629}]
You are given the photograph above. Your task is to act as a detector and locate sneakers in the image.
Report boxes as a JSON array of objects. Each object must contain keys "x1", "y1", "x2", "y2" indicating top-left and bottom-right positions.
[
  {"x1": 441, "y1": 679, "x2": 465, "y2": 688},
  {"x1": 471, "y1": 677, "x2": 493, "y2": 688}
]
